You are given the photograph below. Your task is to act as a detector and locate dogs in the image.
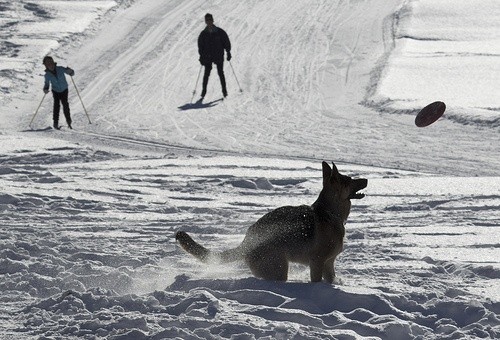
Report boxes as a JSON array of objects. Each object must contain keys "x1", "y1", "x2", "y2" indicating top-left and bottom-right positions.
[{"x1": 175, "y1": 160, "x2": 368, "y2": 283}]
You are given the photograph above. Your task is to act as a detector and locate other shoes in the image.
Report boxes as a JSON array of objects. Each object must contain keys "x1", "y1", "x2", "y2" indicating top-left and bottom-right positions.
[
  {"x1": 66, "y1": 118, "x2": 73, "y2": 129},
  {"x1": 200, "y1": 89, "x2": 206, "y2": 97},
  {"x1": 222, "y1": 90, "x2": 228, "y2": 98},
  {"x1": 53, "y1": 120, "x2": 58, "y2": 128}
]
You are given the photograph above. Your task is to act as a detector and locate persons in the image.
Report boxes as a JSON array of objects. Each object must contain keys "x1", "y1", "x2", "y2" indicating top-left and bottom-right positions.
[
  {"x1": 42, "y1": 55, "x2": 74, "y2": 130},
  {"x1": 198, "y1": 13, "x2": 232, "y2": 99}
]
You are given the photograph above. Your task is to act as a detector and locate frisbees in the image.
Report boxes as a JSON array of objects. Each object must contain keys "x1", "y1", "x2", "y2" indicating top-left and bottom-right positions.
[{"x1": 414, "y1": 100, "x2": 447, "y2": 128}]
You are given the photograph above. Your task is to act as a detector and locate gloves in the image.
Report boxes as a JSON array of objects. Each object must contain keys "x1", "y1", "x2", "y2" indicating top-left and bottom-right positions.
[
  {"x1": 66, "y1": 67, "x2": 74, "y2": 75},
  {"x1": 199, "y1": 58, "x2": 207, "y2": 65},
  {"x1": 227, "y1": 53, "x2": 232, "y2": 61},
  {"x1": 43, "y1": 87, "x2": 49, "y2": 93}
]
